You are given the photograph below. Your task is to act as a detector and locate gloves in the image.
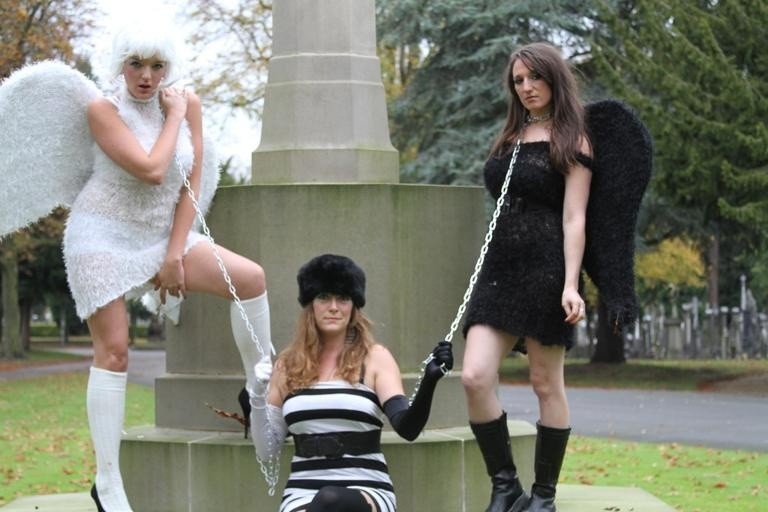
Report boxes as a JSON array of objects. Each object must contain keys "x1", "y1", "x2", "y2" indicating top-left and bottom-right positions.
[
  {"x1": 382, "y1": 341, "x2": 453, "y2": 441},
  {"x1": 249, "y1": 356, "x2": 272, "y2": 409}
]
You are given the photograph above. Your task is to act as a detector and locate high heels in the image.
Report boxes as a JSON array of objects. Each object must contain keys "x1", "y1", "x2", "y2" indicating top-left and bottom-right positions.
[
  {"x1": 238, "y1": 387, "x2": 291, "y2": 439},
  {"x1": 91, "y1": 484, "x2": 105, "y2": 512}
]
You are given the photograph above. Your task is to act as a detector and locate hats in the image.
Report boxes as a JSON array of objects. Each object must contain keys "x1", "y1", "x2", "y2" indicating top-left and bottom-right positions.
[{"x1": 297, "y1": 254, "x2": 365, "y2": 309}]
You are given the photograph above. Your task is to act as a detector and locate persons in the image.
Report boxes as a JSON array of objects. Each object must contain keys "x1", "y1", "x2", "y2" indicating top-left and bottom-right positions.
[
  {"x1": 459, "y1": 42, "x2": 595, "y2": 512},
  {"x1": 248, "y1": 254, "x2": 454, "y2": 511},
  {"x1": 62, "y1": 21, "x2": 272, "y2": 512}
]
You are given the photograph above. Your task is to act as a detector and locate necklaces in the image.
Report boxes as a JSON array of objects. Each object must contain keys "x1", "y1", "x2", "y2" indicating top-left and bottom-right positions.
[{"x1": 525, "y1": 109, "x2": 554, "y2": 124}]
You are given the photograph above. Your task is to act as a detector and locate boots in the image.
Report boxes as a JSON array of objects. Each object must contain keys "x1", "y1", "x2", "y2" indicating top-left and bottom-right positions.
[{"x1": 468, "y1": 409, "x2": 571, "y2": 512}]
